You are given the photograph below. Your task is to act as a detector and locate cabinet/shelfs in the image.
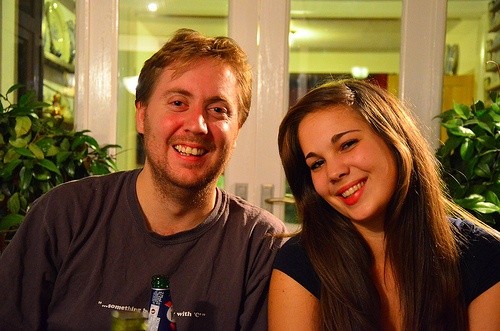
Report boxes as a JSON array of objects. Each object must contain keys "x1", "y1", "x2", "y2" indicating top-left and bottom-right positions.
[{"x1": 387, "y1": 73, "x2": 475, "y2": 156}]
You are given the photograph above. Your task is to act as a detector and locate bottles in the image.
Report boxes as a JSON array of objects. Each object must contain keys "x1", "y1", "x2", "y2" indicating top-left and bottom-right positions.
[{"x1": 146, "y1": 275, "x2": 178, "y2": 331}]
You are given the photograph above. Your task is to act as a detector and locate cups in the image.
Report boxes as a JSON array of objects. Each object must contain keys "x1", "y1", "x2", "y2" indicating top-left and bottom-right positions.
[{"x1": 110, "y1": 309, "x2": 147, "y2": 331}]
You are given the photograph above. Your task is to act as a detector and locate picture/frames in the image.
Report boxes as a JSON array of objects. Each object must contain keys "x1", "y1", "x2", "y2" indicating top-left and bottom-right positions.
[{"x1": 39, "y1": 0, "x2": 77, "y2": 73}]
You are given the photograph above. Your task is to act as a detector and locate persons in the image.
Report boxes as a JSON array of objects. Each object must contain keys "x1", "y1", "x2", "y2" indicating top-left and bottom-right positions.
[
  {"x1": 266, "y1": 78, "x2": 500, "y2": 331},
  {"x1": 0, "y1": 29, "x2": 289, "y2": 331}
]
